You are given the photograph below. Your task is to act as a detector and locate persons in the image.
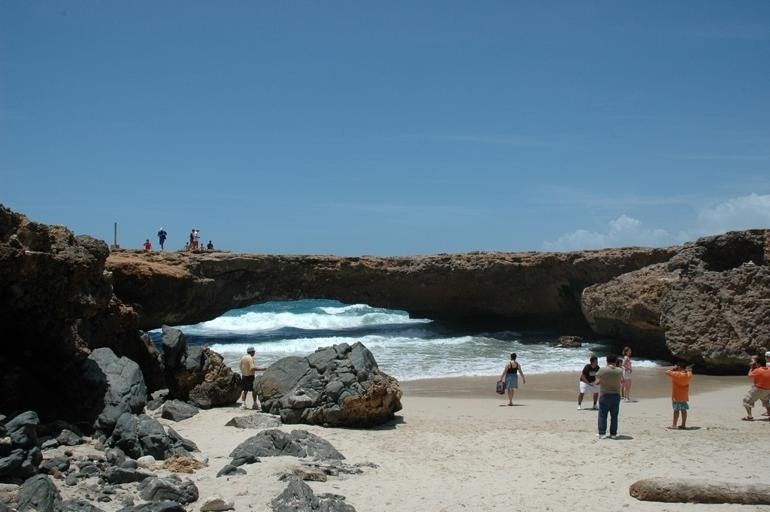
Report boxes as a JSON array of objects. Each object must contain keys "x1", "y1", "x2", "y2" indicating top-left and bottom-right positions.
[
  {"x1": 594, "y1": 353, "x2": 624, "y2": 438},
  {"x1": 207, "y1": 241, "x2": 213, "y2": 249},
  {"x1": 157, "y1": 228, "x2": 168, "y2": 250},
  {"x1": 741, "y1": 357, "x2": 770, "y2": 421},
  {"x1": 183, "y1": 242, "x2": 189, "y2": 249},
  {"x1": 761, "y1": 351, "x2": 770, "y2": 415},
  {"x1": 239, "y1": 346, "x2": 268, "y2": 410},
  {"x1": 189, "y1": 229, "x2": 195, "y2": 250},
  {"x1": 194, "y1": 229, "x2": 200, "y2": 248},
  {"x1": 499, "y1": 352, "x2": 526, "y2": 406},
  {"x1": 664, "y1": 359, "x2": 693, "y2": 429},
  {"x1": 144, "y1": 239, "x2": 152, "y2": 251},
  {"x1": 621, "y1": 346, "x2": 633, "y2": 400},
  {"x1": 577, "y1": 356, "x2": 601, "y2": 410},
  {"x1": 617, "y1": 358, "x2": 623, "y2": 397},
  {"x1": 200, "y1": 243, "x2": 205, "y2": 250}
]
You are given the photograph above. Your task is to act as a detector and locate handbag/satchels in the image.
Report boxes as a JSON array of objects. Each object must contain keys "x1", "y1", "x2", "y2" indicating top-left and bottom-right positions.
[{"x1": 496, "y1": 381, "x2": 506, "y2": 394}]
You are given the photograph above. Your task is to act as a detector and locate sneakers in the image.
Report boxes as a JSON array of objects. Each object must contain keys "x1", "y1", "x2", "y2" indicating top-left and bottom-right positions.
[
  {"x1": 241, "y1": 403, "x2": 260, "y2": 410},
  {"x1": 596, "y1": 432, "x2": 620, "y2": 439},
  {"x1": 577, "y1": 405, "x2": 582, "y2": 410},
  {"x1": 593, "y1": 404, "x2": 600, "y2": 410}
]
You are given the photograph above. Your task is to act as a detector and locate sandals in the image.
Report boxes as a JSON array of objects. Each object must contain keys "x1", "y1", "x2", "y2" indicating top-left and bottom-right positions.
[
  {"x1": 741, "y1": 416, "x2": 754, "y2": 421},
  {"x1": 761, "y1": 411, "x2": 770, "y2": 416}
]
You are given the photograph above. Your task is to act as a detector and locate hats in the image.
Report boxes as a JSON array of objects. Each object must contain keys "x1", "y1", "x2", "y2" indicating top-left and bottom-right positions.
[
  {"x1": 765, "y1": 351, "x2": 770, "y2": 357},
  {"x1": 247, "y1": 347, "x2": 256, "y2": 353}
]
